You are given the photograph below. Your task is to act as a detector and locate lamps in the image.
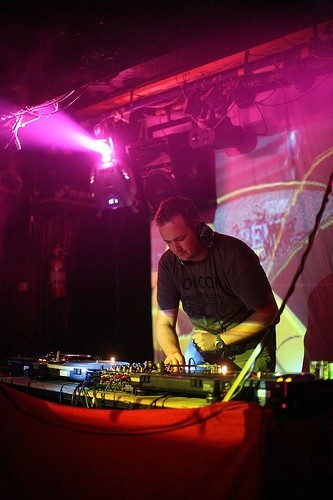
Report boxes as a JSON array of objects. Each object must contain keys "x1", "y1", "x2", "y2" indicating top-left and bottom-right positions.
[
  {"x1": 199, "y1": 76, "x2": 233, "y2": 114},
  {"x1": 184, "y1": 83, "x2": 215, "y2": 128},
  {"x1": 187, "y1": 116, "x2": 246, "y2": 150},
  {"x1": 284, "y1": 46, "x2": 316, "y2": 93},
  {"x1": 223, "y1": 65, "x2": 257, "y2": 110},
  {"x1": 89, "y1": 111, "x2": 147, "y2": 205}
]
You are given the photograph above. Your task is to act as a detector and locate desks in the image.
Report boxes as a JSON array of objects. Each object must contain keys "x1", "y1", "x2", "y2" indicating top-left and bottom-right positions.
[{"x1": 0, "y1": 376, "x2": 333, "y2": 500}]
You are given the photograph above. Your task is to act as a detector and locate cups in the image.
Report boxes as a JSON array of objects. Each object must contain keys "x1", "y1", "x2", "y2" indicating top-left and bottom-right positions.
[{"x1": 310, "y1": 361, "x2": 327, "y2": 380}]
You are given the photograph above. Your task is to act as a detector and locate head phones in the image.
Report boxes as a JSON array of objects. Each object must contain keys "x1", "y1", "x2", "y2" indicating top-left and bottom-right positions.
[{"x1": 198, "y1": 223, "x2": 215, "y2": 248}]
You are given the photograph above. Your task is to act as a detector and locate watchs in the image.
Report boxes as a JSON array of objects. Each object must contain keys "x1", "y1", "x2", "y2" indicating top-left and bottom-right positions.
[{"x1": 215, "y1": 334, "x2": 225, "y2": 351}]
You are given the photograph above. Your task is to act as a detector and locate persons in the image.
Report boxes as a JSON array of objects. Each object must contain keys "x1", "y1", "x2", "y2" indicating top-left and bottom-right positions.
[{"x1": 155, "y1": 196, "x2": 281, "y2": 373}]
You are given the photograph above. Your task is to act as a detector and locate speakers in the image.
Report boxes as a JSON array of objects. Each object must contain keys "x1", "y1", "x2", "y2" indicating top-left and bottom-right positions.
[{"x1": 126, "y1": 127, "x2": 218, "y2": 217}]
